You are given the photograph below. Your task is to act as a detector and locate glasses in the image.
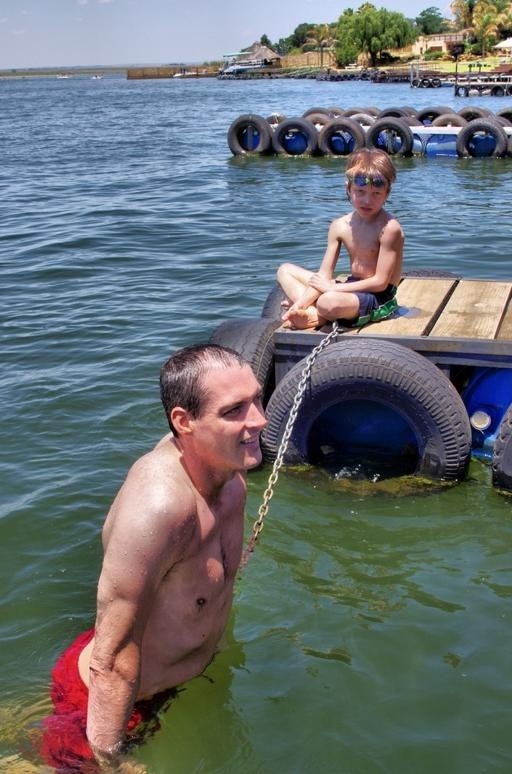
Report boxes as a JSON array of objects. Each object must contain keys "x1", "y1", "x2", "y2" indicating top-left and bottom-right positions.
[{"x1": 349, "y1": 174, "x2": 387, "y2": 186}]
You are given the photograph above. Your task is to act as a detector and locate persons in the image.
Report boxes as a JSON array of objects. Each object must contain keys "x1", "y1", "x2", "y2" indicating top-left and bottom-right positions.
[
  {"x1": 273, "y1": 145, "x2": 407, "y2": 331},
  {"x1": 0, "y1": 340, "x2": 272, "y2": 774}
]
